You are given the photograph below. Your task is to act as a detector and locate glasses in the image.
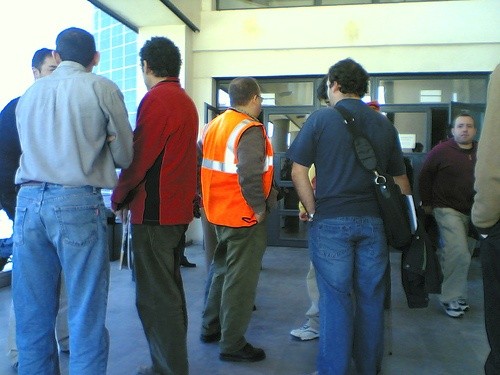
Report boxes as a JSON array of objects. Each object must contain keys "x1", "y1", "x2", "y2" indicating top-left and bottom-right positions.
[{"x1": 258, "y1": 95, "x2": 264, "y2": 102}]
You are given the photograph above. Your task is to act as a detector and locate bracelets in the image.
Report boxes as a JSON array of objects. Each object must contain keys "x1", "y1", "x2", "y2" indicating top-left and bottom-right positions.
[{"x1": 307, "y1": 212, "x2": 315, "y2": 219}]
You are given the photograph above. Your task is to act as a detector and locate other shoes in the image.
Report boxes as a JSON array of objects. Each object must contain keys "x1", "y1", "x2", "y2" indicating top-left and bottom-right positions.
[
  {"x1": 180, "y1": 256, "x2": 196, "y2": 267},
  {"x1": 220, "y1": 343, "x2": 266, "y2": 362},
  {"x1": 200, "y1": 334, "x2": 219, "y2": 341}
]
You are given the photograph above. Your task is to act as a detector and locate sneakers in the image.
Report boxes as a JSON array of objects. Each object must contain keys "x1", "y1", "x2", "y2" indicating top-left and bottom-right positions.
[
  {"x1": 459, "y1": 303, "x2": 470, "y2": 311},
  {"x1": 442, "y1": 301, "x2": 464, "y2": 317},
  {"x1": 290, "y1": 324, "x2": 321, "y2": 340}
]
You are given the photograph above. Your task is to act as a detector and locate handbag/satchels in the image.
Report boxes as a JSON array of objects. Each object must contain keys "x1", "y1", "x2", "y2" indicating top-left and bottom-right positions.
[{"x1": 374, "y1": 175, "x2": 409, "y2": 250}]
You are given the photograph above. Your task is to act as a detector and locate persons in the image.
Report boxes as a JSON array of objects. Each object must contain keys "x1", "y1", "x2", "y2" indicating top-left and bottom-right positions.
[
  {"x1": 290, "y1": 76, "x2": 334, "y2": 341},
  {"x1": 12, "y1": 27, "x2": 135, "y2": 375},
  {"x1": 285, "y1": 58, "x2": 410, "y2": 375},
  {"x1": 470, "y1": 61, "x2": 500, "y2": 375},
  {"x1": 412, "y1": 143, "x2": 423, "y2": 152},
  {"x1": 418, "y1": 113, "x2": 478, "y2": 317},
  {"x1": 111, "y1": 35, "x2": 199, "y2": 375},
  {"x1": 0, "y1": 47, "x2": 72, "y2": 372},
  {"x1": 201, "y1": 76, "x2": 273, "y2": 362}
]
